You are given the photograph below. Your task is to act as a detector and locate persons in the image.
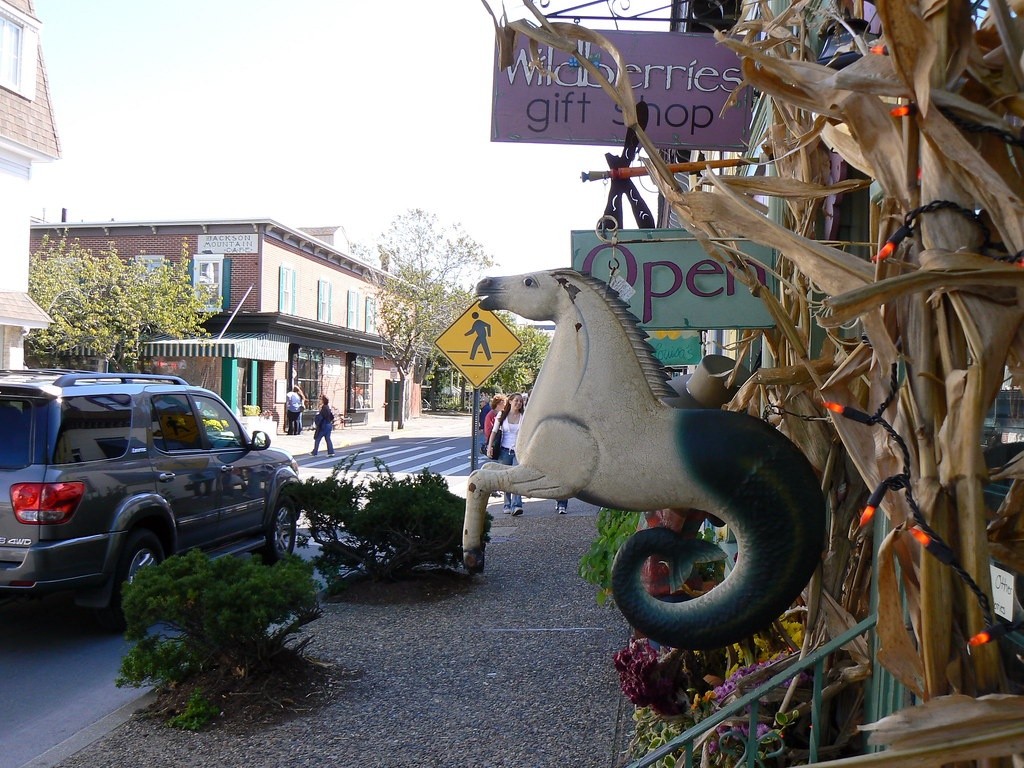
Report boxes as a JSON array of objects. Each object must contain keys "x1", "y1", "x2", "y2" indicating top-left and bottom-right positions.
[
  {"x1": 486, "y1": 391, "x2": 525, "y2": 515},
  {"x1": 553, "y1": 498, "x2": 568, "y2": 515},
  {"x1": 308, "y1": 395, "x2": 335, "y2": 458},
  {"x1": 468, "y1": 397, "x2": 492, "y2": 460},
  {"x1": 483, "y1": 394, "x2": 508, "y2": 442},
  {"x1": 287, "y1": 386, "x2": 305, "y2": 436}
]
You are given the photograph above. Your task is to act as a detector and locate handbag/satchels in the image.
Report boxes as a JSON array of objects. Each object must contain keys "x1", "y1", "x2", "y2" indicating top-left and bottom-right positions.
[
  {"x1": 480, "y1": 410, "x2": 502, "y2": 460},
  {"x1": 300, "y1": 405, "x2": 304, "y2": 412}
]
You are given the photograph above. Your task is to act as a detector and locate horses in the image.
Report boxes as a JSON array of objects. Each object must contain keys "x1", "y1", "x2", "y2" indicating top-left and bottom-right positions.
[{"x1": 458, "y1": 268, "x2": 827, "y2": 651}]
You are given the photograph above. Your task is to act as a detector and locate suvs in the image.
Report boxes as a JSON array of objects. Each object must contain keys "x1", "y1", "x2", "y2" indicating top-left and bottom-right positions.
[{"x1": 0, "y1": 365, "x2": 302, "y2": 636}]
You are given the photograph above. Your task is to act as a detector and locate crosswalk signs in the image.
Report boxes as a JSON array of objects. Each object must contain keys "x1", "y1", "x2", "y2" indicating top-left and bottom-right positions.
[{"x1": 435, "y1": 299, "x2": 521, "y2": 391}]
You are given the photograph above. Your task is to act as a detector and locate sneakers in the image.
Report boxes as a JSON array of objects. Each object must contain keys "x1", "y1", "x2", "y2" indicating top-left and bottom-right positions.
[
  {"x1": 503, "y1": 506, "x2": 511, "y2": 514},
  {"x1": 559, "y1": 507, "x2": 567, "y2": 514},
  {"x1": 555, "y1": 502, "x2": 559, "y2": 510},
  {"x1": 511, "y1": 507, "x2": 523, "y2": 515}
]
